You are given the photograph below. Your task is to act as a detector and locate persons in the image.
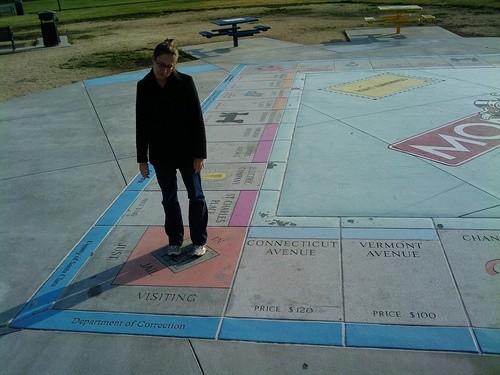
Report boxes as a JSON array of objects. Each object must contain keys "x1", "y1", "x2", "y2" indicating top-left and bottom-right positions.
[{"x1": 136, "y1": 38, "x2": 209, "y2": 258}]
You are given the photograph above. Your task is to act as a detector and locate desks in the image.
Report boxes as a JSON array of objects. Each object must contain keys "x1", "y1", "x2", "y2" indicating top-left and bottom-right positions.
[
  {"x1": 210, "y1": 16, "x2": 259, "y2": 47},
  {"x1": 375, "y1": 5, "x2": 423, "y2": 34}
]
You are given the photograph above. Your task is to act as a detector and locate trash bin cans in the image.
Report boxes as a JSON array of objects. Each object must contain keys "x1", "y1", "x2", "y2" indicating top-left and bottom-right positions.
[{"x1": 38, "y1": 9, "x2": 58, "y2": 46}]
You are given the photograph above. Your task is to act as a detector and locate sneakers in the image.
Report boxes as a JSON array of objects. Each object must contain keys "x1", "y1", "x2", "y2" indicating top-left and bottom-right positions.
[
  {"x1": 167, "y1": 245, "x2": 182, "y2": 256},
  {"x1": 192, "y1": 244, "x2": 207, "y2": 256}
]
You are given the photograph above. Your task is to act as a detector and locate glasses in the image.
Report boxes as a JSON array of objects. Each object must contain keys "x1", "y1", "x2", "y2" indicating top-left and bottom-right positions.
[{"x1": 154, "y1": 58, "x2": 173, "y2": 69}]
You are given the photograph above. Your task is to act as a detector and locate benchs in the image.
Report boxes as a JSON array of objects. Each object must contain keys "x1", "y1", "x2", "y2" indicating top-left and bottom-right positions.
[
  {"x1": 0, "y1": 26, "x2": 16, "y2": 52},
  {"x1": 211, "y1": 27, "x2": 241, "y2": 32},
  {"x1": 422, "y1": 14, "x2": 435, "y2": 22},
  {"x1": 379, "y1": 14, "x2": 411, "y2": 19},
  {"x1": 199, "y1": 31, "x2": 213, "y2": 38},
  {"x1": 227, "y1": 30, "x2": 261, "y2": 37},
  {"x1": 386, "y1": 18, "x2": 420, "y2": 26},
  {"x1": 253, "y1": 26, "x2": 270, "y2": 32},
  {"x1": 364, "y1": 17, "x2": 376, "y2": 24}
]
